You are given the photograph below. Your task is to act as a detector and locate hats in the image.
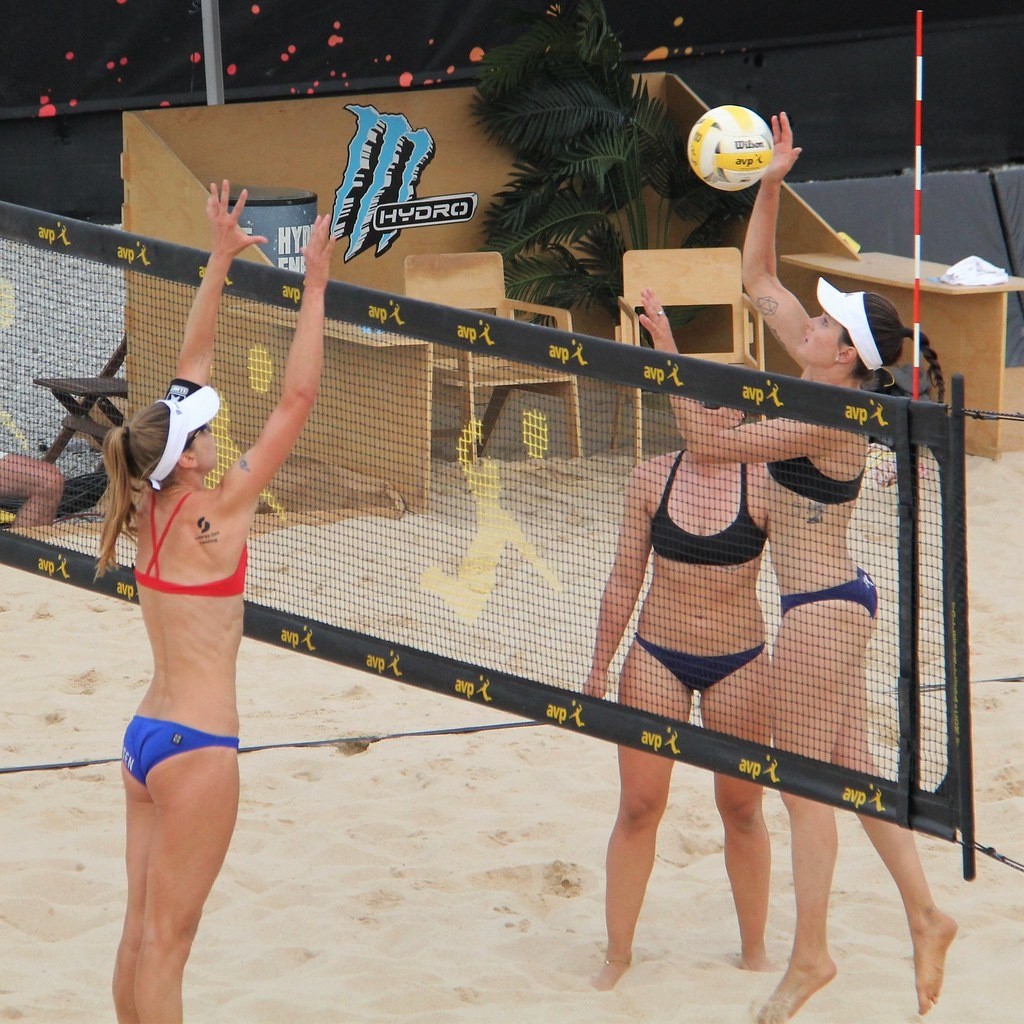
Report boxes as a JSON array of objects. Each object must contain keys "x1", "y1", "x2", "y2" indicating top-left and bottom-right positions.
[
  {"x1": 145, "y1": 385, "x2": 220, "y2": 491},
  {"x1": 817, "y1": 277, "x2": 883, "y2": 371}
]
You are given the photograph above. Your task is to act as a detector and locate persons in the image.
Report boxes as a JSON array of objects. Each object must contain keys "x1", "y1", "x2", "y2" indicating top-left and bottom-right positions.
[
  {"x1": 0, "y1": 451, "x2": 65, "y2": 529},
  {"x1": 582, "y1": 396, "x2": 773, "y2": 991},
  {"x1": 93, "y1": 179, "x2": 336, "y2": 1024},
  {"x1": 638, "y1": 110, "x2": 958, "y2": 1024}
]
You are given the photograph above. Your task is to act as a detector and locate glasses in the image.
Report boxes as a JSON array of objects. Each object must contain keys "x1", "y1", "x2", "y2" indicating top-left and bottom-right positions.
[
  {"x1": 183, "y1": 423, "x2": 212, "y2": 452},
  {"x1": 701, "y1": 401, "x2": 720, "y2": 410}
]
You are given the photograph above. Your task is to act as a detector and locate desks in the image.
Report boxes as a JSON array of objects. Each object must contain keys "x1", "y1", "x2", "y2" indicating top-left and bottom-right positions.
[{"x1": 779, "y1": 247, "x2": 1024, "y2": 462}]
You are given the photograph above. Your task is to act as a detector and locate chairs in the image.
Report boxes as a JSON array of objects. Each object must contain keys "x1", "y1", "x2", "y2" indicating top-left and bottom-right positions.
[
  {"x1": 614, "y1": 245, "x2": 767, "y2": 472},
  {"x1": 31, "y1": 330, "x2": 128, "y2": 469},
  {"x1": 402, "y1": 249, "x2": 583, "y2": 467}
]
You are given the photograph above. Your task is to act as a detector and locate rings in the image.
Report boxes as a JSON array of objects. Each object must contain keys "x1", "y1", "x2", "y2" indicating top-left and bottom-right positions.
[{"x1": 658, "y1": 310, "x2": 663, "y2": 318}]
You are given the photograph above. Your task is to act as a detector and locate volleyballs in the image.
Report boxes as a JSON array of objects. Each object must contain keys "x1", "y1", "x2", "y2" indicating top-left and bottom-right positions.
[{"x1": 687, "y1": 105, "x2": 774, "y2": 191}]
